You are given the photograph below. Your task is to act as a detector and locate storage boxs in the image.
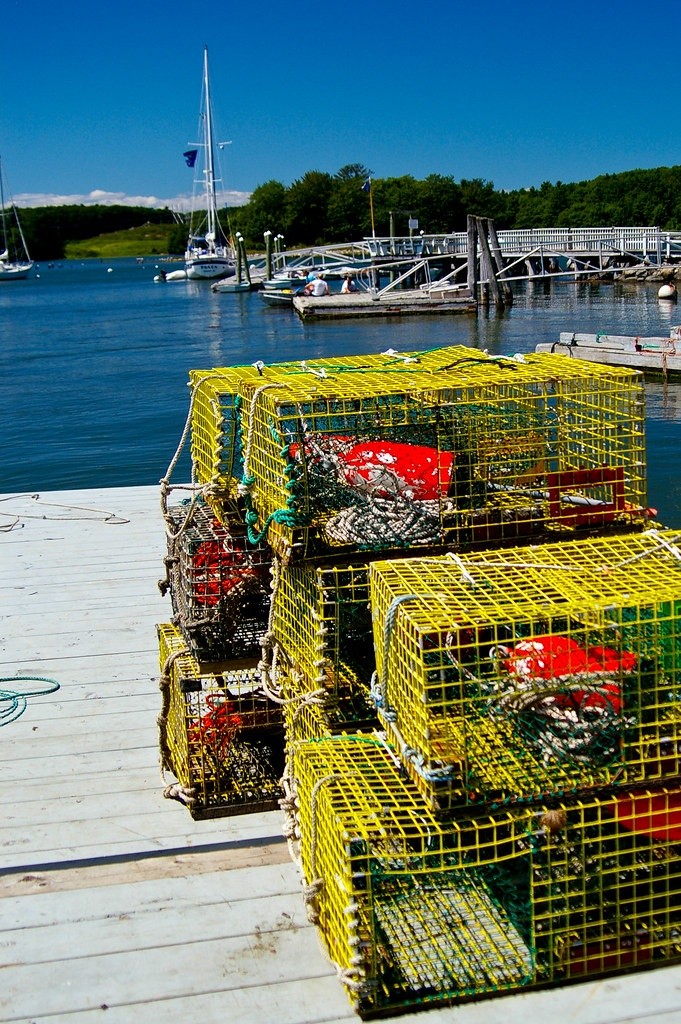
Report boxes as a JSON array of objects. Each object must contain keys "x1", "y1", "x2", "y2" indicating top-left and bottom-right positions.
[{"x1": 154, "y1": 343, "x2": 679, "y2": 1021}]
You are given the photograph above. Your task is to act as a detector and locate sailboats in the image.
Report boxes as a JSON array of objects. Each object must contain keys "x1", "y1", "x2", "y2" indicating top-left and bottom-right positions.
[
  {"x1": 1, "y1": 156, "x2": 34, "y2": 279},
  {"x1": 183, "y1": 45, "x2": 237, "y2": 279}
]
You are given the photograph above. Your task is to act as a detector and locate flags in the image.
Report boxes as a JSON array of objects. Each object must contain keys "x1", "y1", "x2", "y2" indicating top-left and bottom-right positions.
[
  {"x1": 361, "y1": 181, "x2": 369, "y2": 193},
  {"x1": 183, "y1": 149, "x2": 197, "y2": 167}
]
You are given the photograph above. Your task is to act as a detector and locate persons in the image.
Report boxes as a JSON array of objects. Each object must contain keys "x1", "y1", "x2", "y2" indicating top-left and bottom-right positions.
[
  {"x1": 301, "y1": 270, "x2": 317, "y2": 284},
  {"x1": 305, "y1": 273, "x2": 328, "y2": 296},
  {"x1": 339, "y1": 274, "x2": 359, "y2": 293}
]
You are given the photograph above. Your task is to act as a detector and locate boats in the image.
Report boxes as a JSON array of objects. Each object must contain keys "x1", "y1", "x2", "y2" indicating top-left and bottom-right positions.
[{"x1": 211, "y1": 265, "x2": 365, "y2": 306}]
